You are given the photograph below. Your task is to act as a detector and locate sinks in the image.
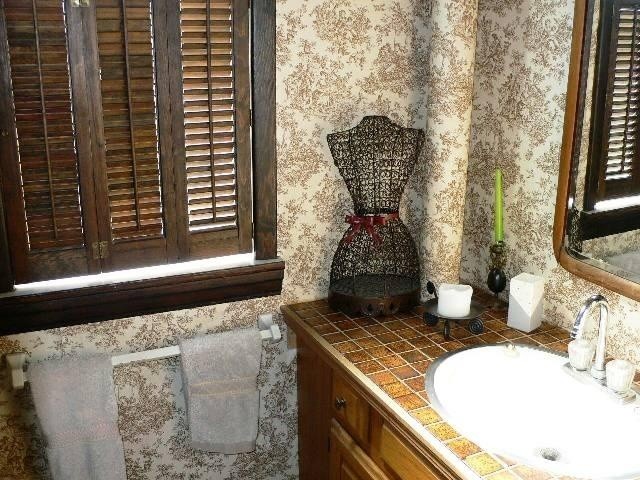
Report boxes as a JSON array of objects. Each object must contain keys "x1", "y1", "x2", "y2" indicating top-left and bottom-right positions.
[{"x1": 569, "y1": 293, "x2": 607, "y2": 380}]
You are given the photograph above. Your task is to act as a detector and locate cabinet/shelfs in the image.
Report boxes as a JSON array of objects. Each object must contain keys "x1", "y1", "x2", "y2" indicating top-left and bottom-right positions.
[{"x1": 295, "y1": 337, "x2": 441, "y2": 479}]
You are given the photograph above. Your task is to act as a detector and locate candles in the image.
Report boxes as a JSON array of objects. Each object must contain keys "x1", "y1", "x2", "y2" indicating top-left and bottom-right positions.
[{"x1": 494, "y1": 166, "x2": 504, "y2": 242}]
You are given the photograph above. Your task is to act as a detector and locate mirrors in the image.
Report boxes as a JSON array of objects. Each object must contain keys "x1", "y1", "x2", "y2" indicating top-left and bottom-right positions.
[{"x1": 551, "y1": 0, "x2": 639, "y2": 303}]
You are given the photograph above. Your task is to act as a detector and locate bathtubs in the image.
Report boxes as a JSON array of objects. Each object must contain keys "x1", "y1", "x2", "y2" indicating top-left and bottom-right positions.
[{"x1": 423, "y1": 342, "x2": 640, "y2": 479}]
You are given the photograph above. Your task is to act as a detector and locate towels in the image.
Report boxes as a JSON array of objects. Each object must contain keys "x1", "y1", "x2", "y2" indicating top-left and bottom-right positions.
[
  {"x1": 27, "y1": 351, "x2": 127, "y2": 480},
  {"x1": 176, "y1": 326, "x2": 263, "y2": 455}
]
